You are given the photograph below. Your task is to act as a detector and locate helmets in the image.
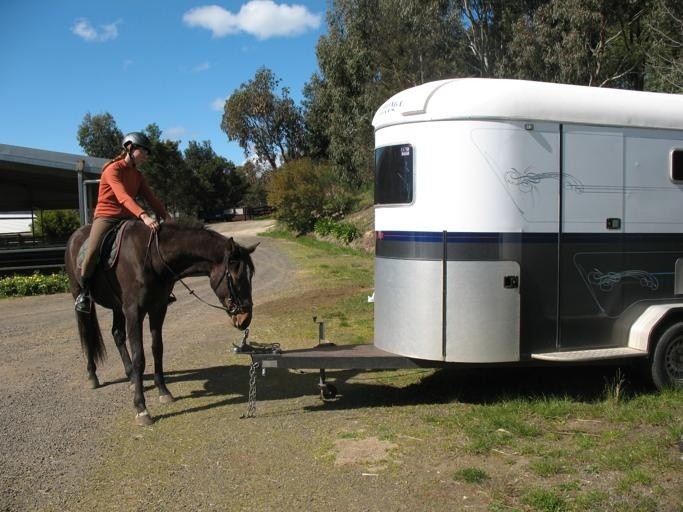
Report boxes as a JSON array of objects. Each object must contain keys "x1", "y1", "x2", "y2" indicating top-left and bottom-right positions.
[{"x1": 122, "y1": 132, "x2": 151, "y2": 150}]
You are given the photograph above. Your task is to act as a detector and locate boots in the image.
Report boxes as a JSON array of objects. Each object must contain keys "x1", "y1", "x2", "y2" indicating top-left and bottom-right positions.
[{"x1": 77, "y1": 277, "x2": 92, "y2": 310}]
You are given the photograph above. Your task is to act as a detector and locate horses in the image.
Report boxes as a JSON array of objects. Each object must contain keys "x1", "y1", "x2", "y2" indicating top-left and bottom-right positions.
[{"x1": 63, "y1": 211, "x2": 262, "y2": 427}]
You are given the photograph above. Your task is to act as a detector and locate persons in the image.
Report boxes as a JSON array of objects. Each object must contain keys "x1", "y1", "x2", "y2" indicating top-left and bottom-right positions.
[{"x1": 76, "y1": 132, "x2": 177, "y2": 313}]
